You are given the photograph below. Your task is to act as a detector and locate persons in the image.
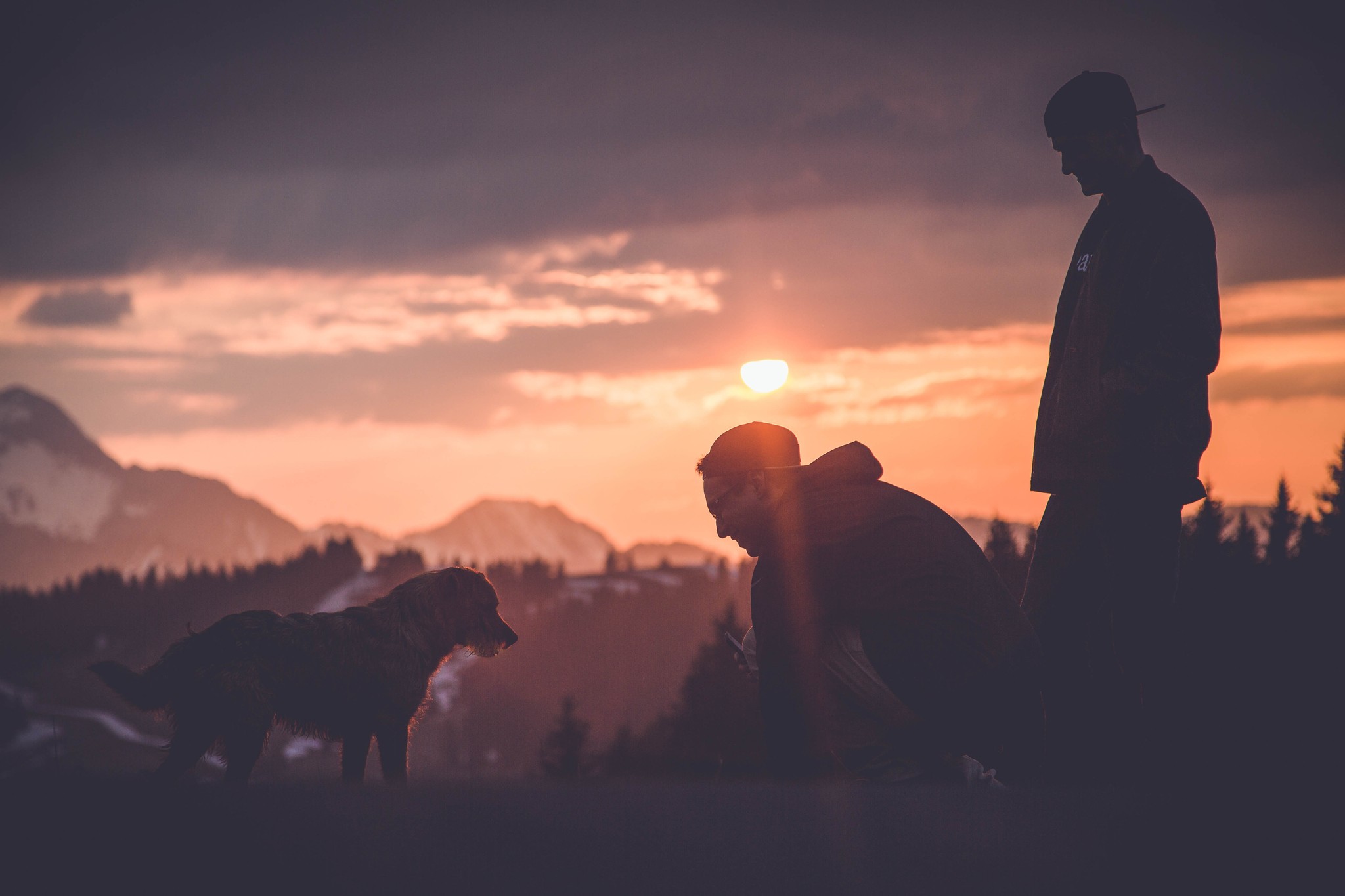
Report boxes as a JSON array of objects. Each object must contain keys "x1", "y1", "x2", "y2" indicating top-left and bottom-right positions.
[
  {"x1": 1019, "y1": 72, "x2": 1222, "y2": 787},
  {"x1": 696, "y1": 422, "x2": 1046, "y2": 786}
]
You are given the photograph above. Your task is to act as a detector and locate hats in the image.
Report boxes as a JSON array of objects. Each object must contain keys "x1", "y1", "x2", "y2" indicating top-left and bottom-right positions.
[
  {"x1": 709, "y1": 421, "x2": 802, "y2": 467},
  {"x1": 1041, "y1": 73, "x2": 1168, "y2": 139}
]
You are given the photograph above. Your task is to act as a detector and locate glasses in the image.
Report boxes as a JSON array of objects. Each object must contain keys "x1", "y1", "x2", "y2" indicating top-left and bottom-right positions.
[{"x1": 709, "y1": 472, "x2": 749, "y2": 521}]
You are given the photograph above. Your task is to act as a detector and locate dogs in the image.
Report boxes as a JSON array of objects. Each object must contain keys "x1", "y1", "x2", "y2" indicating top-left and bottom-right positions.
[{"x1": 88, "y1": 568, "x2": 516, "y2": 784}]
